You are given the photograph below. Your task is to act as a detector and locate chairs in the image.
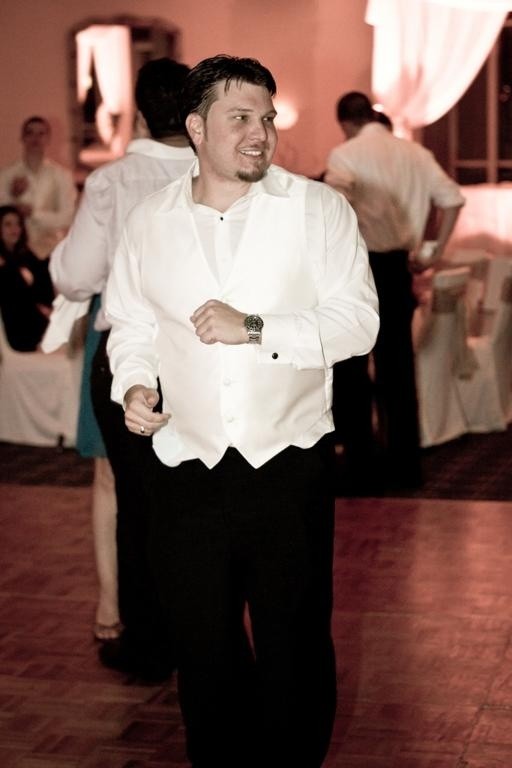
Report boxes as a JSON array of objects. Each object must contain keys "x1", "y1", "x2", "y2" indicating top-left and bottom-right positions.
[
  {"x1": 2, "y1": 292, "x2": 86, "y2": 446},
  {"x1": 410, "y1": 263, "x2": 511, "y2": 445}
]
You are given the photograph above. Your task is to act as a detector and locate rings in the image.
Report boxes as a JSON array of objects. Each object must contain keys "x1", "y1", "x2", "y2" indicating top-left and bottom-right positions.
[{"x1": 139, "y1": 425, "x2": 146, "y2": 435}]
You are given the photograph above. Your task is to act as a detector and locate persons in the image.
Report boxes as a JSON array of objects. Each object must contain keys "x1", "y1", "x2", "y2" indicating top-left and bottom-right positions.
[
  {"x1": 321, "y1": 88, "x2": 470, "y2": 501},
  {"x1": 0, "y1": 115, "x2": 80, "y2": 265},
  {"x1": 91, "y1": 50, "x2": 382, "y2": 768},
  {"x1": 371, "y1": 112, "x2": 446, "y2": 241},
  {"x1": 0, "y1": 201, "x2": 90, "y2": 356},
  {"x1": 37, "y1": 278, "x2": 124, "y2": 650},
  {"x1": 48, "y1": 55, "x2": 199, "y2": 689}
]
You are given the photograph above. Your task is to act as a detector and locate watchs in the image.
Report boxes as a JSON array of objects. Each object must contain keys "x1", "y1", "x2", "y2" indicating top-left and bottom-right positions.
[{"x1": 241, "y1": 313, "x2": 266, "y2": 346}]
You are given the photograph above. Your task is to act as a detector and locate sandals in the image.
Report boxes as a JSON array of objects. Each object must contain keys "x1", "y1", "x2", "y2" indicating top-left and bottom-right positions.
[{"x1": 93, "y1": 603, "x2": 122, "y2": 642}]
